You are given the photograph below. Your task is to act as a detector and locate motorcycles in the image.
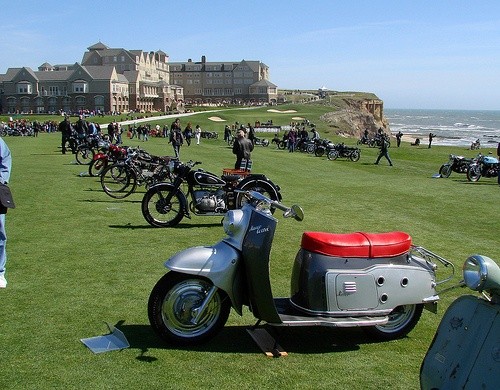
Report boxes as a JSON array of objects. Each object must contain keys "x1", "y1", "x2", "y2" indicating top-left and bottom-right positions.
[
  {"x1": 419, "y1": 255, "x2": 500, "y2": 390},
  {"x1": 466, "y1": 150, "x2": 500, "y2": 182},
  {"x1": 439, "y1": 154, "x2": 478, "y2": 179},
  {"x1": 357, "y1": 135, "x2": 371, "y2": 145},
  {"x1": 141, "y1": 149, "x2": 283, "y2": 224},
  {"x1": 471, "y1": 141, "x2": 481, "y2": 150},
  {"x1": 65, "y1": 119, "x2": 360, "y2": 199},
  {"x1": 369, "y1": 133, "x2": 391, "y2": 148}
]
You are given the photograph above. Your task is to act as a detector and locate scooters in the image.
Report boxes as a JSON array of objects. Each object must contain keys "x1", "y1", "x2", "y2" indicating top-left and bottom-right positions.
[{"x1": 147, "y1": 188, "x2": 455, "y2": 345}]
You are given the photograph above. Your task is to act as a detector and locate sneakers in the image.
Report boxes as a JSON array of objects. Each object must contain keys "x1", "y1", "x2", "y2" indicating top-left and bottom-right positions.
[{"x1": 0, "y1": 275, "x2": 7, "y2": 288}]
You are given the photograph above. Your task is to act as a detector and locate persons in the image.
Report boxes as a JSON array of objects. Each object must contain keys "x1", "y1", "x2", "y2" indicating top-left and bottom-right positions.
[
  {"x1": 396, "y1": 131, "x2": 403, "y2": 148},
  {"x1": 233, "y1": 130, "x2": 253, "y2": 172},
  {"x1": 223, "y1": 121, "x2": 256, "y2": 148},
  {"x1": 168, "y1": 118, "x2": 202, "y2": 161},
  {"x1": 0, "y1": 133, "x2": 16, "y2": 289},
  {"x1": 0, "y1": 110, "x2": 63, "y2": 137},
  {"x1": 428, "y1": 132, "x2": 436, "y2": 148},
  {"x1": 127, "y1": 121, "x2": 169, "y2": 142},
  {"x1": 378, "y1": 126, "x2": 383, "y2": 134},
  {"x1": 363, "y1": 127, "x2": 369, "y2": 138},
  {"x1": 373, "y1": 136, "x2": 393, "y2": 166},
  {"x1": 59, "y1": 106, "x2": 124, "y2": 154},
  {"x1": 264, "y1": 119, "x2": 321, "y2": 153},
  {"x1": 122, "y1": 108, "x2": 208, "y2": 122},
  {"x1": 495, "y1": 142, "x2": 500, "y2": 185}
]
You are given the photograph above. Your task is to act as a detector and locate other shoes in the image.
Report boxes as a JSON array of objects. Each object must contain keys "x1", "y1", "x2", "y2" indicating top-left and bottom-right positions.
[
  {"x1": 428, "y1": 147, "x2": 432, "y2": 148},
  {"x1": 59, "y1": 141, "x2": 125, "y2": 155},
  {"x1": 5, "y1": 129, "x2": 60, "y2": 138},
  {"x1": 130, "y1": 136, "x2": 168, "y2": 141},
  {"x1": 289, "y1": 150, "x2": 295, "y2": 153},
  {"x1": 396, "y1": 145, "x2": 400, "y2": 147},
  {"x1": 388, "y1": 164, "x2": 394, "y2": 166},
  {"x1": 187, "y1": 143, "x2": 200, "y2": 148},
  {"x1": 374, "y1": 162, "x2": 379, "y2": 165}
]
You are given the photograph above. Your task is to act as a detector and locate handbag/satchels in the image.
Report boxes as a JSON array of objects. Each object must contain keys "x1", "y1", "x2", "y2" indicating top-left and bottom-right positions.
[{"x1": 0, "y1": 183, "x2": 15, "y2": 214}]
